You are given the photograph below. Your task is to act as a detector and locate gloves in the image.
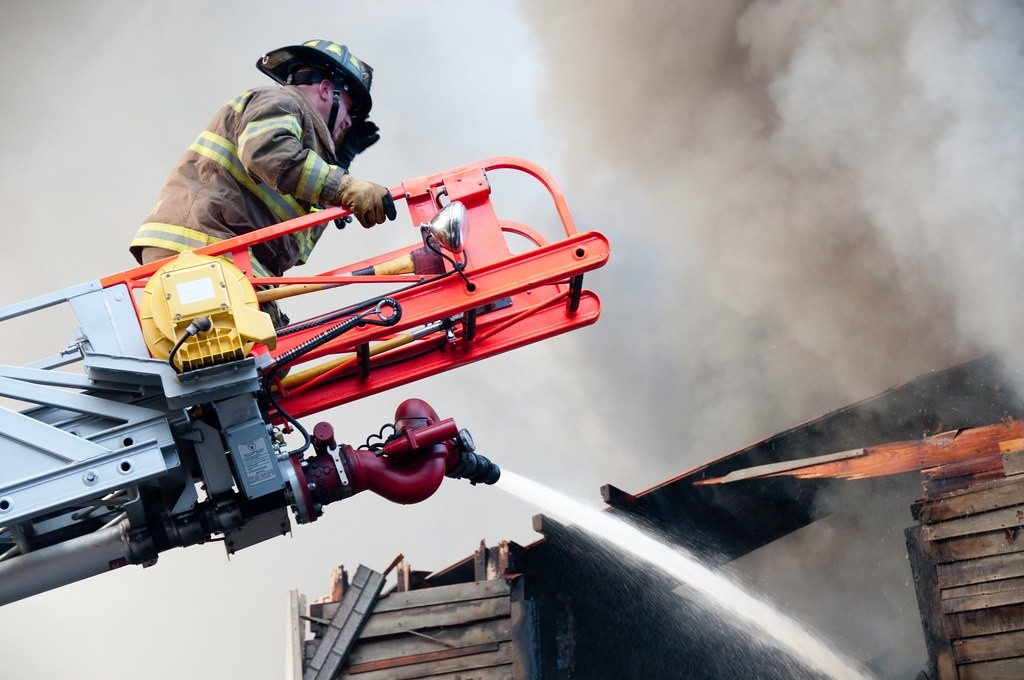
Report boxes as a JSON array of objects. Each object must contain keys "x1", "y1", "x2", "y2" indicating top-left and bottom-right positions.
[
  {"x1": 332, "y1": 173, "x2": 397, "y2": 229},
  {"x1": 335, "y1": 120, "x2": 380, "y2": 170}
]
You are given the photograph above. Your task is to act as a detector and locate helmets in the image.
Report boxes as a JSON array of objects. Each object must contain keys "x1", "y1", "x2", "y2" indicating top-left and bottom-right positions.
[{"x1": 256, "y1": 39, "x2": 372, "y2": 120}]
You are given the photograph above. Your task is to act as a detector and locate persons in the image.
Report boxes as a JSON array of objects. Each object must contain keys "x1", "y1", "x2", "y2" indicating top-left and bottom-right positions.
[{"x1": 129, "y1": 40, "x2": 397, "y2": 384}]
